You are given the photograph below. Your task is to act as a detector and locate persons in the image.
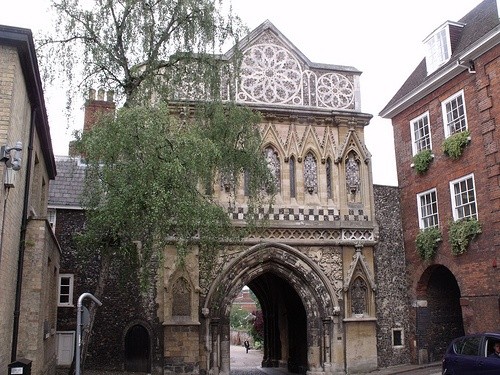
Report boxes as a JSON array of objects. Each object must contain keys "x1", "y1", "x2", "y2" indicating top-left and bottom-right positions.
[
  {"x1": 488, "y1": 342, "x2": 500, "y2": 359},
  {"x1": 244, "y1": 339, "x2": 251, "y2": 353}
]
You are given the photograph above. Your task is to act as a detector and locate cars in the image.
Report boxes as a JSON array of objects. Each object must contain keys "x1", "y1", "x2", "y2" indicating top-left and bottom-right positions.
[{"x1": 441, "y1": 332, "x2": 500, "y2": 374}]
